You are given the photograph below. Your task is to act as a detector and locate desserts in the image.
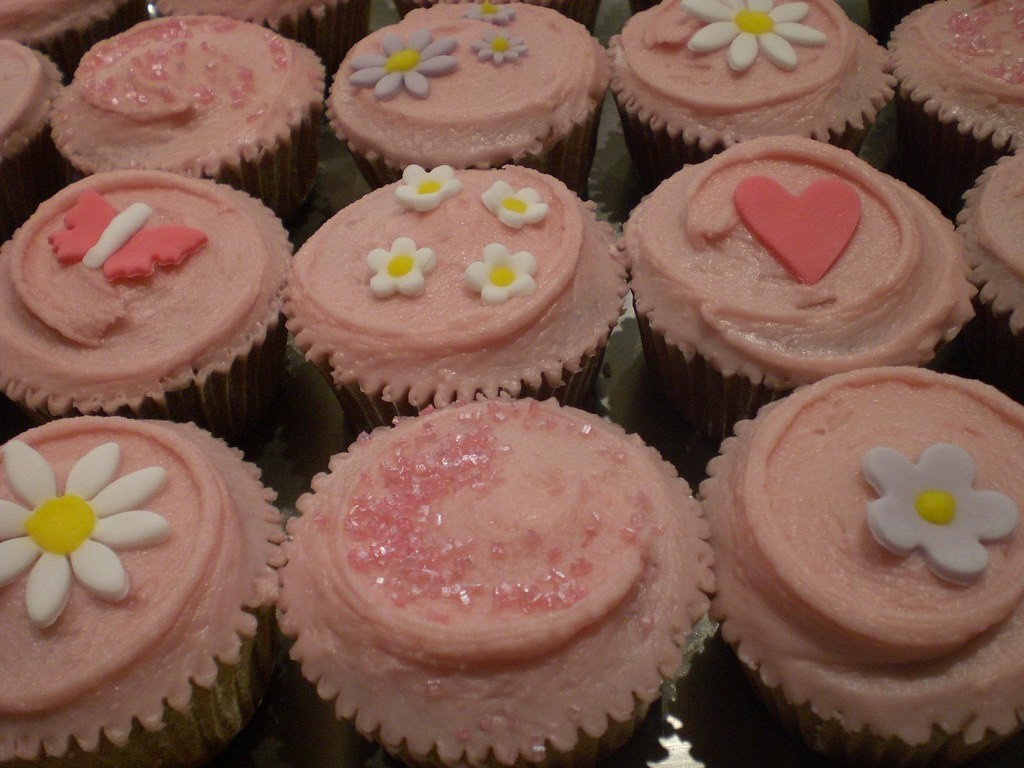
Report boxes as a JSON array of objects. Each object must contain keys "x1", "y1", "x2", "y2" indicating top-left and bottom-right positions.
[{"x1": 0, "y1": 0, "x2": 1024, "y2": 768}]
